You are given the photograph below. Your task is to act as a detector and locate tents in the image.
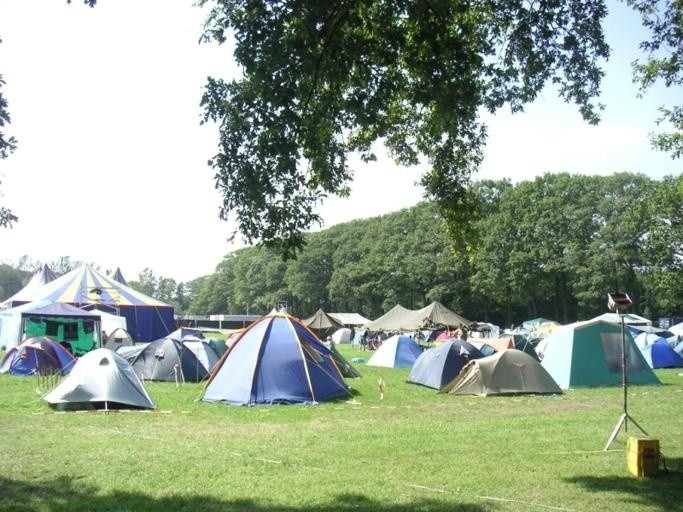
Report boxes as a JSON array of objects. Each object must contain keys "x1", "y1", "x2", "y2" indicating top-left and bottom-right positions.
[
  {"x1": 0, "y1": 260, "x2": 683, "y2": 396},
  {"x1": 41, "y1": 349, "x2": 153, "y2": 410},
  {"x1": 200, "y1": 312, "x2": 356, "y2": 407}
]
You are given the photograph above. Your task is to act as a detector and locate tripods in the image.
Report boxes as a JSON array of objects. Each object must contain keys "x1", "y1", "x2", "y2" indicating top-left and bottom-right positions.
[{"x1": 604, "y1": 316, "x2": 649, "y2": 451}]
